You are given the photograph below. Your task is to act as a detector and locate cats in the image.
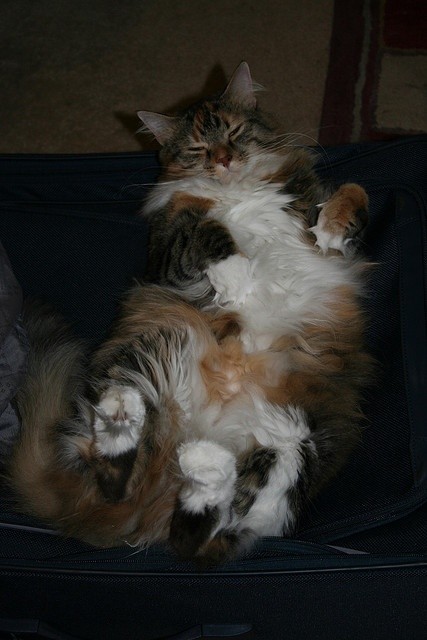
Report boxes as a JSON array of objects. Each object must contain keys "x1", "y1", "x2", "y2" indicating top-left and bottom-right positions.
[{"x1": 14, "y1": 59, "x2": 372, "y2": 558}]
